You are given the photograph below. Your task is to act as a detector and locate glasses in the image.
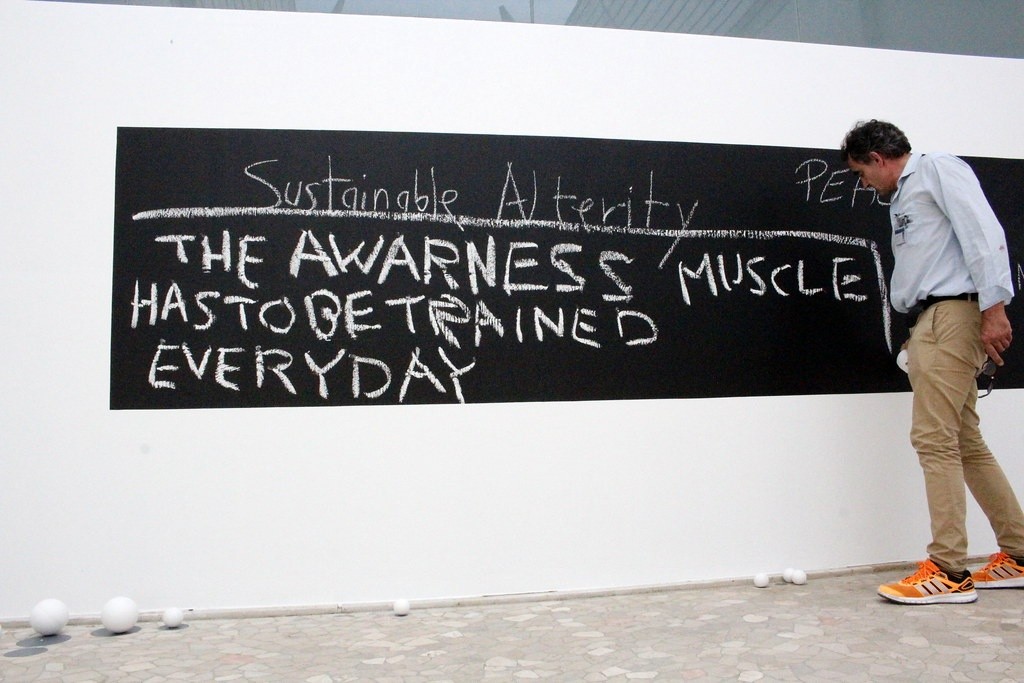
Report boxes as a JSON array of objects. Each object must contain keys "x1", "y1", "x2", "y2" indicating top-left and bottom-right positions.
[{"x1": 976, "y1": 356, "x2": 995, "y2": 399}]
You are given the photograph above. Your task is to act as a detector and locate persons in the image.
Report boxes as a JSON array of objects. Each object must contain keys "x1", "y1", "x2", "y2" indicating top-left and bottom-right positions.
[{"x1": 839, "y1": 116, "x2": 1024, "y2": 607}]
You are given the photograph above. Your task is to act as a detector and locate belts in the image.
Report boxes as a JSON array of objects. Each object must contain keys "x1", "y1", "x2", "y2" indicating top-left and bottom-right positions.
[{"x1": 909, "y1": 293, "x2": 979, "y2": 326}]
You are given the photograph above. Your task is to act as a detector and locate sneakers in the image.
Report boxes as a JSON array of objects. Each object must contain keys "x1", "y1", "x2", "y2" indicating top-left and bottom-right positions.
[
  {"x1": 878, "y1": 558, "x2": 978, "y2": 604},
  {"x1": 972, "y1": 551, "x2": 1024, "y2": 588}
]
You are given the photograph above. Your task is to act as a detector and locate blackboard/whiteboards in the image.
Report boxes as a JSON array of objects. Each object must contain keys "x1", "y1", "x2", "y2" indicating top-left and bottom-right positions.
[{"x1": 108, "y1": 128, "x2": 1024, "y2": 409}]
